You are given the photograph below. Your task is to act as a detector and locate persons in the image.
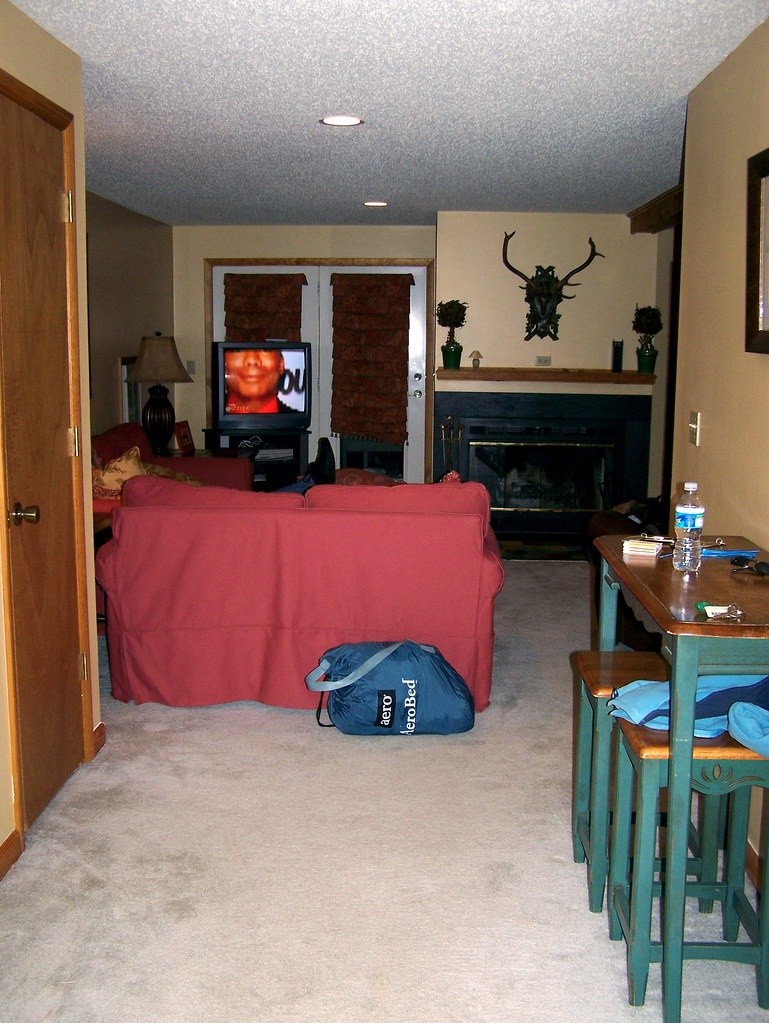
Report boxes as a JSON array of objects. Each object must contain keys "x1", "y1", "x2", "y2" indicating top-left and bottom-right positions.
[
  {"x1": 273, "y1": 436, "x2": 336, "y2": 498},
  {"x1": 221, "y1": 345, "x2": 297, "y2": 412}
]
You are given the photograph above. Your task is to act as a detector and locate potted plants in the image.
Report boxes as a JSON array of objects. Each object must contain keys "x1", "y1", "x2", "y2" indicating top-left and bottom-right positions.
[
  {"x1": 631, "y1": 302, "x2": 664, "y2": 375},
  {"x1": 436, "y1": 300, "x2": 470, "y2": 370}
]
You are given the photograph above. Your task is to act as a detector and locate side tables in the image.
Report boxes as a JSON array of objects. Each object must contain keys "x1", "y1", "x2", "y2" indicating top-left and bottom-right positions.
[{"x1": 92, "y1": 512, "x2": 113, "y2": 624}]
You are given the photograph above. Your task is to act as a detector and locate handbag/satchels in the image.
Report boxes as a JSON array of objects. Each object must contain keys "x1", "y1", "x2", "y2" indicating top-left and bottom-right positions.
[{"x1": 306, "y1": 640, "x2": 475, "y2": 735}]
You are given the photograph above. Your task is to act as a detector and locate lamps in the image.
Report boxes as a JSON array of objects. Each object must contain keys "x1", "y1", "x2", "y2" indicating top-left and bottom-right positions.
[{"x1": 124, "y1": 336, "x2": 194, "y2": 458}]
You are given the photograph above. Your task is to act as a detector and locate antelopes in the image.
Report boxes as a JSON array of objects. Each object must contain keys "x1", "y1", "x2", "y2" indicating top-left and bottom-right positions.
[{"x1": 501, "y1": 229, "x2": 607, "y2": 320}]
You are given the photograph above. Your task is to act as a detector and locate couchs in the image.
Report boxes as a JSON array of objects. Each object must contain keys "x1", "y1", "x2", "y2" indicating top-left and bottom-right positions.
[
  {"x1": 95, "y1": 475, "x2": 506, "y2": 713},
  {"x1": 91, "y1": 422, "x2": 254, "y2": 634}
]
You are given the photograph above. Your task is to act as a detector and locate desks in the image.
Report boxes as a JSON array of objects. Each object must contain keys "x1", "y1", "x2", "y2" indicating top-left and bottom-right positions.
[{"x1": 595, "y1": 534, "x2": 769, "y2": 1023}]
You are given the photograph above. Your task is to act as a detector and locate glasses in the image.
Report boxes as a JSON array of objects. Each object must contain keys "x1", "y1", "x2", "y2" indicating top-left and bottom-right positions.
[{"x1": 730, "y1": 556, "x2": 769, "y2": 575}]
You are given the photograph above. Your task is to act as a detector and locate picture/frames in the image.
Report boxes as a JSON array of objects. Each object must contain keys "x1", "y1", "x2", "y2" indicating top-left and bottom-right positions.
[
  {"x1": 117, "y1": 356, "x2": 141, "y2": 425},
  {"x1": 745, "y1": 147, "x2": 769, "y2": 355},
  {"x1": 173, "y1": 420, "x2": 196, "y2": 453}
]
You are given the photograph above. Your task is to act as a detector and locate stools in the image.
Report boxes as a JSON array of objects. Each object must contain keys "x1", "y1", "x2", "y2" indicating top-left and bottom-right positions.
[
  {"x1": 608, "y1": 718, "x2": 769, "y2": 1011},
  {"x1": 569, "y1": 651, "x2": 721, "y2": 913}
]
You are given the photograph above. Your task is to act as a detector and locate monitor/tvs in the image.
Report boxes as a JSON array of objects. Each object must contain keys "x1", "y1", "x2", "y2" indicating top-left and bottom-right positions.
[{"x1": 211, "y1": 341, "x2": 312, "y2": 430}]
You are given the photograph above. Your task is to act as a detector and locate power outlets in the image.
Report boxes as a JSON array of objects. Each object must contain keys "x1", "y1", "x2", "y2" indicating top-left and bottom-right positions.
[{"x1": 534, "y1": 356, "x2": 551, "y2": 366}]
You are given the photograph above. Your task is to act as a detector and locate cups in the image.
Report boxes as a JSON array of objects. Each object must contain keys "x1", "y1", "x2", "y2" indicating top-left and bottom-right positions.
[{"x1": 611, "y1": 341, "x2": 623, "y2": 373}]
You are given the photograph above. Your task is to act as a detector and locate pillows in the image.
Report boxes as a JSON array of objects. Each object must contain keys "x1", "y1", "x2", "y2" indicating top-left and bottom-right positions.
[{"x1": 93, "y1": 446, "x2": 205, "y2": 496}]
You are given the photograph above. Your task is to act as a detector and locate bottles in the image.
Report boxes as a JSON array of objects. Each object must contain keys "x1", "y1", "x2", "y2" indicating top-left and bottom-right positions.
[{"x1": 672, "y1": 482, "x2": 706, "y2": 571}]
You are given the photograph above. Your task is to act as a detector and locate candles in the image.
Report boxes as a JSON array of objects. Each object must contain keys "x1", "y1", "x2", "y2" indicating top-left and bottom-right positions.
[{"x1": 441, "y1": 426, "x2": 445, "y2": 439}]
